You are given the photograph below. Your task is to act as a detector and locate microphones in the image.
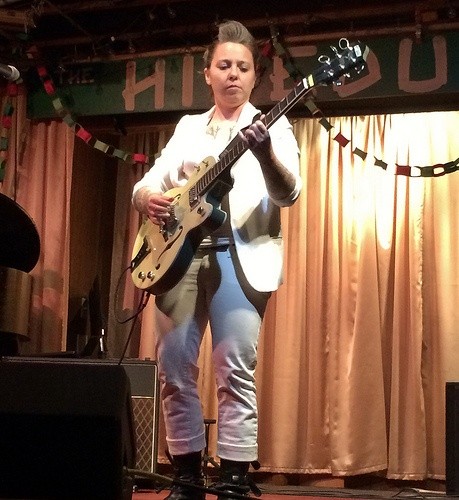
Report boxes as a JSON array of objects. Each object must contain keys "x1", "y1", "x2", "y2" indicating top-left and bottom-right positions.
[{"x1": 0, "y1": 64, "x2": 20, "y2": 82}]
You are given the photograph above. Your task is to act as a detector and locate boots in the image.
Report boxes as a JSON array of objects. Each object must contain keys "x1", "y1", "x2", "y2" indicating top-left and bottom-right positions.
[
  {"x1": 157, "y1": 452, "x2": 208, "y2": 500},
  {"x1": 217, "y1": 457, "x2": 255, "y2": 498}
]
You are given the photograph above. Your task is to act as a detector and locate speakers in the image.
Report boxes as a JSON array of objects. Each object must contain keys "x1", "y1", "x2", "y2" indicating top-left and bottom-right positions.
[
  {"x1": 0, "y1": 359, "x2": 137, "y2": 500},
  {"x1": 445, "y1": 381, "x2": 459, "y2": 496}
]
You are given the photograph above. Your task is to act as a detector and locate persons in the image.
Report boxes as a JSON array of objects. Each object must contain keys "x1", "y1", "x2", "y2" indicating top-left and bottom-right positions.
[{"x1": 130, "y1": 18, "x2": 304, "y2": 500}]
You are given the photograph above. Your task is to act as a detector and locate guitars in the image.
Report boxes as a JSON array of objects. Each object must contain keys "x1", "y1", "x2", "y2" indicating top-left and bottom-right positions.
[{"x1": 130, "y1": 37, "x2": 369, "y2": 297}]
